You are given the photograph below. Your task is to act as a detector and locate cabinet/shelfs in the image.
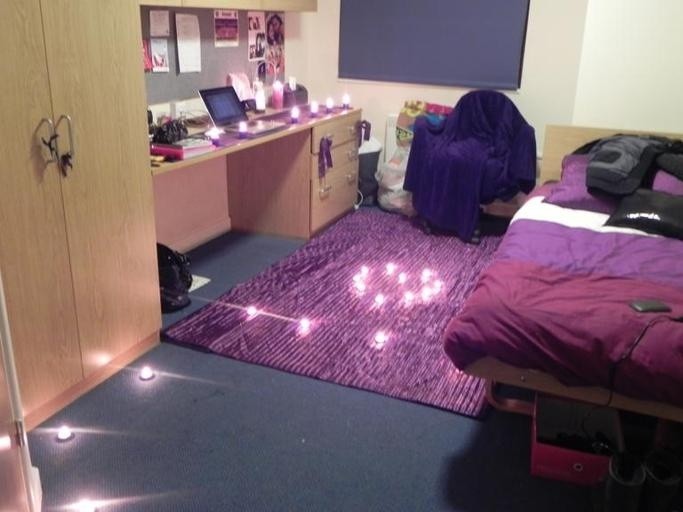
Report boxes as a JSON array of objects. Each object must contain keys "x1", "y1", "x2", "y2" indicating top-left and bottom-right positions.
[
  {"x1": 2, "y1": 2, "x2": 159, "y2": 448},
  {"x1": 310, "y1": 111, "x2": 360, "y2": 241}
]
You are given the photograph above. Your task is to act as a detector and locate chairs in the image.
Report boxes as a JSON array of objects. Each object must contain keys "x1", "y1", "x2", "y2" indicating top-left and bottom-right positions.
[{"x1": 403, "y1": 90, "x2": 535, "y2": 244}]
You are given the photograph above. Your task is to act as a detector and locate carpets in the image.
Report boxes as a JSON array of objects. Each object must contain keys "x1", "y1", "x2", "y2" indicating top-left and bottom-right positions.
[{"x1": 161, "y1": 204, "x2": 506, "y2": 422}]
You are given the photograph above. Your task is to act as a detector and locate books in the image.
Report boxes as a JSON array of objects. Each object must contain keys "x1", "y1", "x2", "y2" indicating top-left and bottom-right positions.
[
  {"x1": 150, "y1": 136, "x2": 215, "y2": 162},
  {"x1": 172, "y1": 137, "x2": 212, "y2": 148}
]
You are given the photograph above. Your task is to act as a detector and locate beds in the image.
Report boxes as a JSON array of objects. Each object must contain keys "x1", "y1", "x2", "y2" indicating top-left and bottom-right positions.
[{"x1": 441, "y1": 124, "x2": 681, "y2": 459}]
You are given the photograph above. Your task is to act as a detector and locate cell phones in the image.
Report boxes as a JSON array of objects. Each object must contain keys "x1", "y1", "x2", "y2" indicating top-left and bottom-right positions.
[{"x1": 629, "y1": 301, "x2": 671, "y2": 312}]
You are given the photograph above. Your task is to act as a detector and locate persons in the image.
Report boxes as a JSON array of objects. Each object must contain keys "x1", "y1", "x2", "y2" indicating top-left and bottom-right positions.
[{"x1": 248, "y1": 13, "x2": 284, "y2": 74}]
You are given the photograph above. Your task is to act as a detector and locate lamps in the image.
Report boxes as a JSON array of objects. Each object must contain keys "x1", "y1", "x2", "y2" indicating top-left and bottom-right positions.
[{"x1": 210, "y1": 82, "x2": 351, "y2": 144}]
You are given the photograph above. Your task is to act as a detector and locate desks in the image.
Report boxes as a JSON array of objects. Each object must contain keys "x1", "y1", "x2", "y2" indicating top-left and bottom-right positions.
[{"x1": 151, "y1": 98, "x2": 359, "y2": 241}]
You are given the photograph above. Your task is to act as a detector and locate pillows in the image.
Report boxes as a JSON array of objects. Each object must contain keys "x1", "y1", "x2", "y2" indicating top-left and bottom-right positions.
[{"x1": 541, "y1": 154, "x2": 681, "y2": 218}]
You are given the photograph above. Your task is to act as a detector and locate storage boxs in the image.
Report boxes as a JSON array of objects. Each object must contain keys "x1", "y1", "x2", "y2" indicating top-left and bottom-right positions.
[{"x1": 529, "y1": 388, "x2": 624, "y2": 489}]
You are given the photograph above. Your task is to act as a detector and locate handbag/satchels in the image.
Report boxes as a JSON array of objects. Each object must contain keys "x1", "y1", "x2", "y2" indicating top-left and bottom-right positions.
[{"x1": 156, "y1": 241, "x2": 192, "y2": 314}]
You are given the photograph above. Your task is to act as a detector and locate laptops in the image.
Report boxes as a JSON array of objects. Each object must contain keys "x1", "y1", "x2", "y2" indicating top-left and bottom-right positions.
[{"x1": 198, "y1": 87, "x2": 286, "y2": 136}]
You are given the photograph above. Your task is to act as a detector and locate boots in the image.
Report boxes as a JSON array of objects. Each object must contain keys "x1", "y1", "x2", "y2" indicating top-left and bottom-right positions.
[{"x1": 606, "y1": 450, "x2": 683, "y2": 512}]
